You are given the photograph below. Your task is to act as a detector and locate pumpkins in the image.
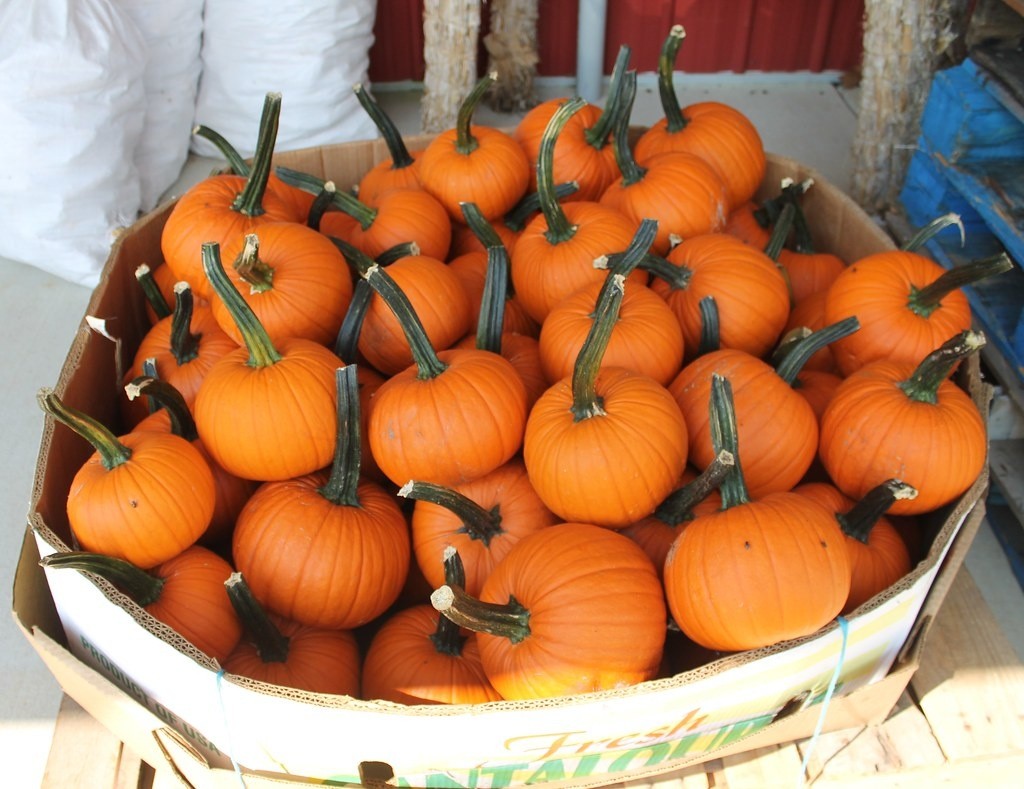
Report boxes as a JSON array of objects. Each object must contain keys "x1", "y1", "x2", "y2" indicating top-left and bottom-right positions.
[{"x1": 35, "y1": 24, "x2": 1014, "y2": 705}]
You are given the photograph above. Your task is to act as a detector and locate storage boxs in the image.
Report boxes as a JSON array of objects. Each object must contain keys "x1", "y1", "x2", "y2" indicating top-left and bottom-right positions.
[{"x1": 13, "y1": 124, "x2": 989, "y2": 789}]
